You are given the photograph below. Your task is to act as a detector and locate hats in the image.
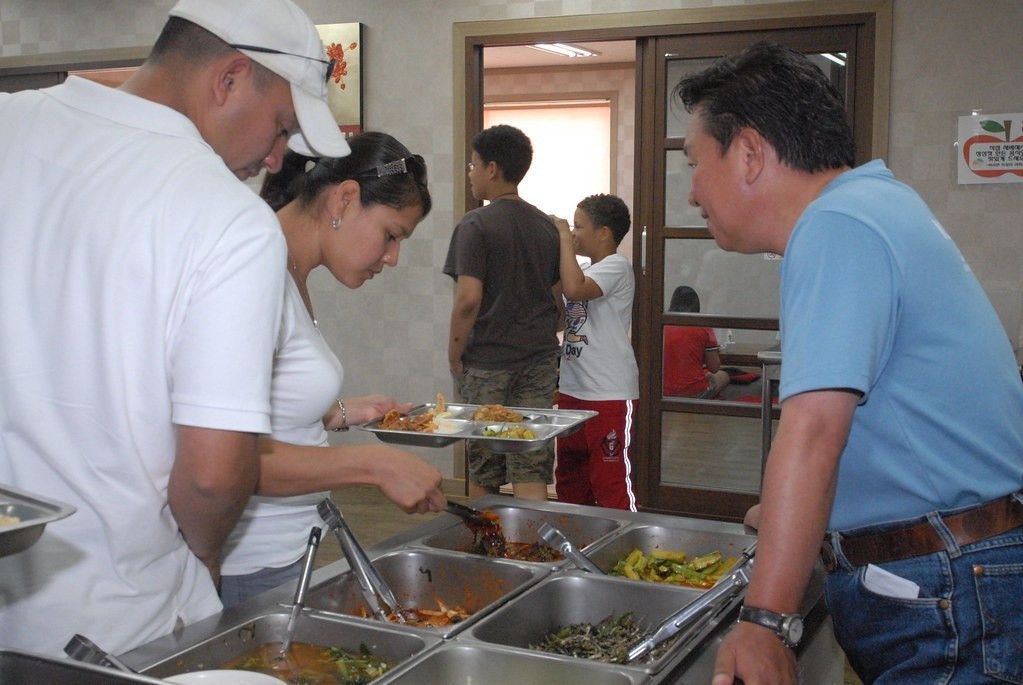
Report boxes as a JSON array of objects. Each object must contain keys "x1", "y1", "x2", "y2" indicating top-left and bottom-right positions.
[{"x1": 168, "y1": 0, "x2": 351, "y2": 158}]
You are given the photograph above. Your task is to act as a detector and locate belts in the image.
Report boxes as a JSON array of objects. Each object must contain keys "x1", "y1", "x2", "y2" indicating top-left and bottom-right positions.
[{"x1": 819, "y1": 488, "x2": 1023, "y2": 573}]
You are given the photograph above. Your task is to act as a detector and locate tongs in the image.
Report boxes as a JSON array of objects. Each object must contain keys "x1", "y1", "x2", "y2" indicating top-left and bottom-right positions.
[
  {"x1": 625, "y1": 567, "x2": 750, "y2": 664},
  {"x1": 316, "y1": 497, "x2": 408, "y2": 624},
  {"x1": 63, "y1": 633, "x2": 139, "y2": 674},
  {"x1": 443, "y1": 499, "x2": 492, "y2": 526},
  {"x1": 537, "y1": 521, "x2": 606, "y2": 575}
]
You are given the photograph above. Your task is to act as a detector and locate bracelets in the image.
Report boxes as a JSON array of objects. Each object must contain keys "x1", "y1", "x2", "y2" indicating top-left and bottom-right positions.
[{"x1": 331, "y1": 398, "x2": 349, "y2": 432}]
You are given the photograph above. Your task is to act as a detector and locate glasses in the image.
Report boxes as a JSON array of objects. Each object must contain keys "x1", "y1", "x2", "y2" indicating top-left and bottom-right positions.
[
  {"x1": 468, "y1": 162, "x2": 490, "y2": 171},
  {"x1": 341, "y1": 155, "x2": 429, "y2": 190},
  {"x1": 229, "y1": 43, "x2": 335, "y2": 84}
]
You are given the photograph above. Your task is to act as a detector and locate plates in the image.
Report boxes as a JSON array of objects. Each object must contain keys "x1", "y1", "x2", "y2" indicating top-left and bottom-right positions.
[{"x1": 159, "y1": 669, "x2": 288, "y2": 685}]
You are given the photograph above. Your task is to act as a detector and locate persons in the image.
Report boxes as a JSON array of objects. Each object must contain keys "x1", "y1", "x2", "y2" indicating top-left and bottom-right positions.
[
  {"x1": 546, "y1": 193, "x2": 639, "y2": 512},
  {"x1": 218, "y1": 131, "x2": 450, "y2": 618},
  {"x1": 0, "y1": 0, "x2": 353, "y2": 685},
  {"x1": 442, "y1": 124, "x2": 564, "y2": 499},
  {"x1": 663, "y1": 286, "x2": 730, "y2": 399},
  {"x1": 672, "y1": 38, "x2": 1023, "y2": 685}
]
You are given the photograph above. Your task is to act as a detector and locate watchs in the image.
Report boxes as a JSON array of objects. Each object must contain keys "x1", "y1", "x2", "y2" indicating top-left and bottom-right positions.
[{"x1": 737, "y1": 605, "x2": 805, "y2": 647}]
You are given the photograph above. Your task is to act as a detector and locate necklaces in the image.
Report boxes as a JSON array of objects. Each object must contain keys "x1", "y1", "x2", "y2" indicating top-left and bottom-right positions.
[
  {"x1": 293, "y1": 263, "x2": 318, "y2": 328},
  {"x1": 490, "y1": 192, "x2": 519, "y2": 202}
]
